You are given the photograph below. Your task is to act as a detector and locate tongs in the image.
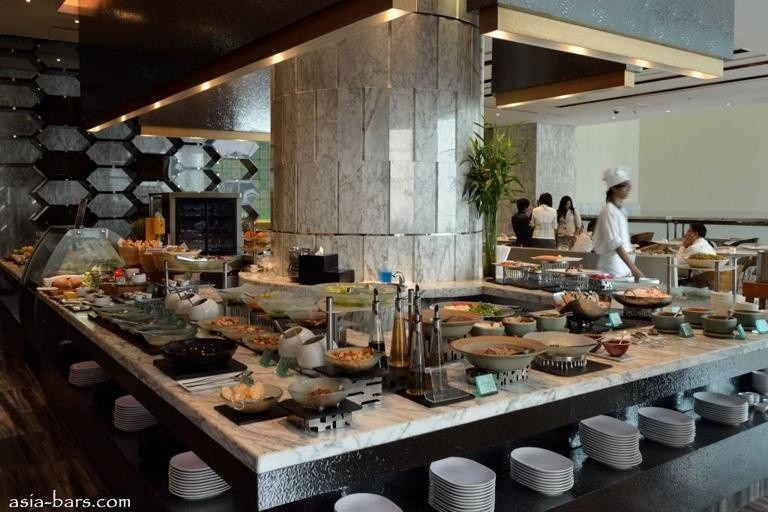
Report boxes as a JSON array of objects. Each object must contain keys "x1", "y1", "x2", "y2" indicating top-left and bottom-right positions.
[
  {"x1": 132, "y1": 327, "x2": 162, "y2": 336},
  {"x1": 347, "y1": 283, "x2": 376, "y2": 295},
  {"x1": 109, "y1": 314, "x2": 143, "y2": 326},
  {"x1": 174, "y1": 255, "x2": 208, "y2": 268}
]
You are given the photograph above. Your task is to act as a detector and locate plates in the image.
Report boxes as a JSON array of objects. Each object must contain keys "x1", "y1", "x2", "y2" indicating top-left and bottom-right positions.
[
  {"x1": 429, "y1": 456, "x2": 497, "y2": 512},
  {"x1": 429, "y1": 301, "x2": 515, "y2": 317},
  {"x1": 244, "y1": 333, "x2": 281, "y2": 351},
  {"x1": 694, "y1": 391, "x2": 748, "y2": 424},
  {"x1": 579, "y1": 415, "x2": 643, "y2": 467},
  {"x1": 510, "y1": 446, "x2": 574, "y2": 494},
  {"x1": 219, "y1": 326, "x2": 270, "y2": 338},
  {"x1": 703, "y1": 331, "x2": 733, "y2": 338},
  {"x1": 742, "y1": 326, "x2": 754, "y2": 330},
  {"x1": 753, "y1": 371, "x2": 768, "y2": 394},
  {"x1": 638, "y1": 407, "x2": 696, "y2": 446},
  {"x1": 114, "y1": 395, "x2": 159, "y2": 432},
  {"x1": 683, "y1": 257, "x2": 729, "y2": 267},
  {"x1": 197, "y1": 316, "x2": 243, "y2": 329},
  {"x1": 168, "y1": 451, "x2": 232, "y2": 499},
  {"x1": 69, "y1": 361, "x2": 105, "y2": 386},
  {"x1": 547, "y1": 268, "x2": 602, "y2": 275},
  {"x1": 334, "y1": 493, "x2": 403, "y2": 512},
  {"x1": 656, "y1": 328, "x2": 678, "y2": 333},
  {"x1": 531, "y1": 256, "x2": 583, "y2": 262}
]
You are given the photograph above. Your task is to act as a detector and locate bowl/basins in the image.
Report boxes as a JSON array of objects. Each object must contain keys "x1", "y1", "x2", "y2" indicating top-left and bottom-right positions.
[
  {"x1": 86, "y1": 289, "x2": 102, "y2": 302},
  {"x1": 534, "y1": 313, "x2": 567, "y2": 330},
  {"x1": 475, "y1": 320, "x2": 504, "y2": 336},
  {"x1": 126, "y1": 268, "x2": 140, "y2": 279},
  {"x1": 652, "y1": 312, "x2": 685, "y2": 327},
  {"x1": 421, "y1": 310, "x2": 485, "y2": 337},
  {"x1": 132, "y1": 273, "x2": 145, "y2": 283},
  {"x1": 288, "y1": 377, "x2": 353, "y2": 407},
  {"x1": 729, "y1": 310, "x2": 762, "y2": 326},
  {"x1": 451, "y1": 336, "x2": 548, "y2": 370},
  {"x1": 161, "y1": 338, "x2": 238, "y2": 366},
  {"x1": 710, "y1": 292, "x2": 744, "y2": 310},
  {"x1": 524, "y1": 332, "x2": 598, "y2": 354},
  {"x1": 221, "y1": 384, "x2": 282, "y2": 412},
  {"x1": 94, "y1": 295, "x2": 111, "y2": 306},
  {"x1": 733, "y1": 301, "x2": 758, "y2": 312},
  {"x1": 584, "y1": 334, "x2": 605, "y2": 353},
  {"x1": 324, "y1": 347, "x2": 382, "y2": 369},
  {"x1": 605, "y1": 341, "x2": 629, "y2": 357},
  {"x1": 502, "y1": 316, "x2": 536, "y2": 335},
  {"x1": 701, "y1": 316, "x2": 737, "y2": 333},
  {"x1": 682, "y1": 307, "x2": 714, "y2": 324}
]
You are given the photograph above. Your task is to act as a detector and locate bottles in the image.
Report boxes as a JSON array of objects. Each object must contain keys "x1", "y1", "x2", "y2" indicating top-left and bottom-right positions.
[
  {"x1": 390, "y1": 287, "x2": 409, "y2": 367},
  {"x1": 411, "y1": 285, "x2": 424, "y2": 323},
  {"x1": 428, "y1": 305, "x2": 447, "y2": 390},
  {"x1": 368, "y1": 290, "x2": 386, "y2": 367},
  {"x1": 406, "y1": 320, "x2": 425, "y2": 395}
]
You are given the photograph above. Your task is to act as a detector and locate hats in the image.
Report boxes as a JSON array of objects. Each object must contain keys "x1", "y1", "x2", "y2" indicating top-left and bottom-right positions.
[{"x1": 602, "y1": 167, "x2": 630, "y2": 188}]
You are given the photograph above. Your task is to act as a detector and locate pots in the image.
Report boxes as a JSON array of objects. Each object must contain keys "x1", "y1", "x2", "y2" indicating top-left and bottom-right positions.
[{"x1": 143, "y1": 328, "x2": 197, "y2": 346}]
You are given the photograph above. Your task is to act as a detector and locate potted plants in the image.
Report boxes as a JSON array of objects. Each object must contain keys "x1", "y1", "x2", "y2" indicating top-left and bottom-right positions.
[{"x1": 459, "y1": 114, "x2": 530, "y2": 282}]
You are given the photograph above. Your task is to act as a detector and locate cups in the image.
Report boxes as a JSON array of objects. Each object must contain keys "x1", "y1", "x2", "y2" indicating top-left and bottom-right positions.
[
  {"x1": 124, "y1": 291, "x2": 152, "y2": 301},
  {"x1": 378, "y1": 261, "x2": 393, "y2": 284},
  {"x1": 162, "y1": 279, "x2": 188, "y2": 287}
]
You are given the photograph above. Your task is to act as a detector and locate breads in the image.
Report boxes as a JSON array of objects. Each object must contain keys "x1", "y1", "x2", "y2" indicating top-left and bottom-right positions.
[{"x1": 51, "y1": 276, "x2": 82, "y2": 292}]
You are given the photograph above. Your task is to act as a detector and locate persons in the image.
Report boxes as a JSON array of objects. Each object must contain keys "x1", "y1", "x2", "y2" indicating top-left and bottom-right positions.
[
  {"x1": 571, "y1": 219, "x2": 598, "y2": 252},
  {"x1": 556, "y1": 195, "x2": 582, "y2": 248},
  {"x1": 529, "y1": 193, "x2": 558, "y2": 249},
  {"x1": 676, "y1": 221, "x2": 716, "y2": 288},
  {"x1": 592, "y1": 166, "x2": 646, "y2": 282},
  {"x1": 512, "y1": 197, "x2": 533, "y2": 247}
]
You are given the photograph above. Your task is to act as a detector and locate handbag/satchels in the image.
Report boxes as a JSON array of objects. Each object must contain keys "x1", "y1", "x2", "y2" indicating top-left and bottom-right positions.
[{"x1": 575, "y1": 223, "x2": 584, "y2": 234}]
